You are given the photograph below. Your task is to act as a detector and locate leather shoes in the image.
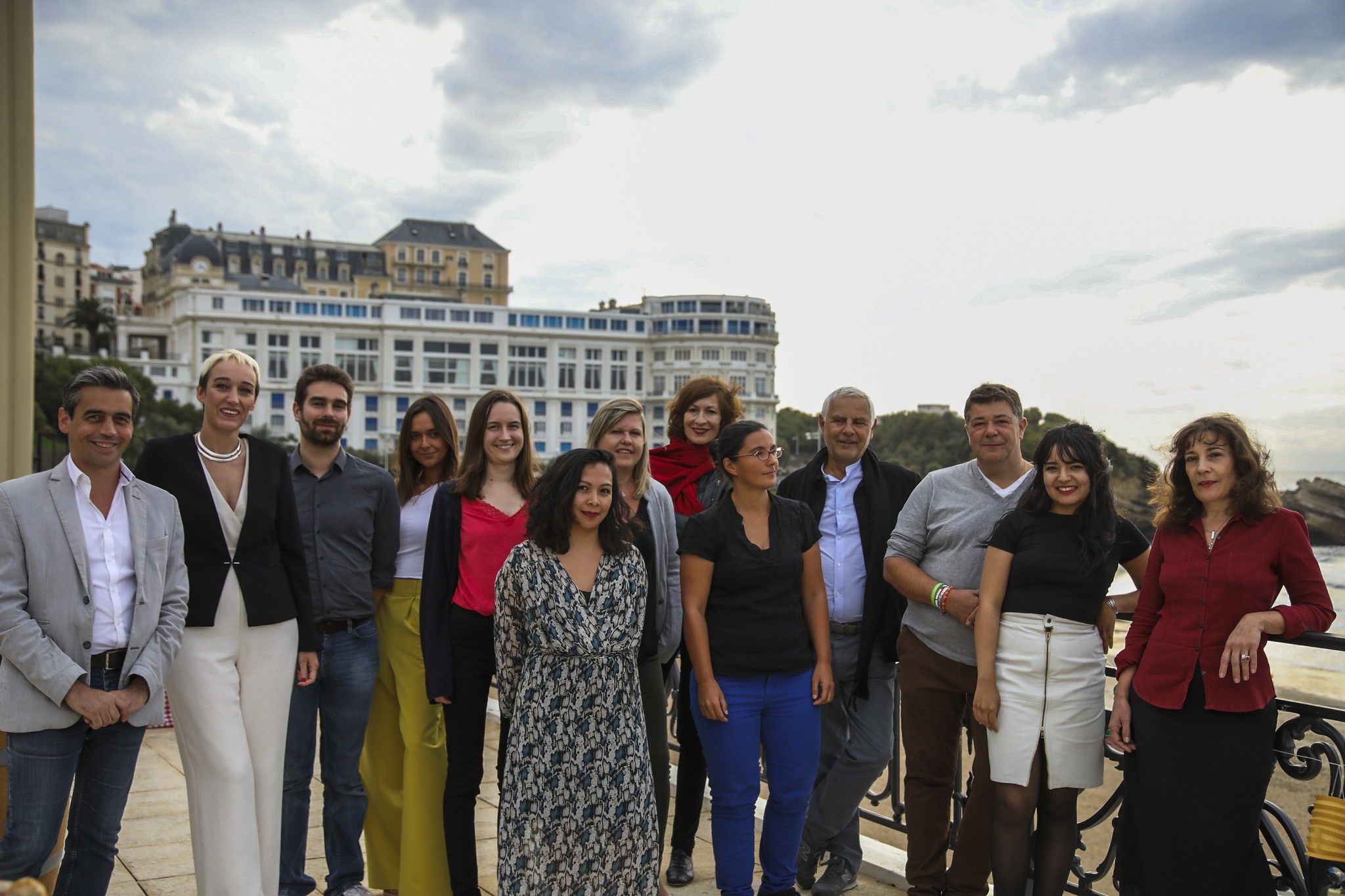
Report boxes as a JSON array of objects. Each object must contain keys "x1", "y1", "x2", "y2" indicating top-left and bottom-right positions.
[{"x1": 666, "y1": 850, "x2": 694, "y2": 886}]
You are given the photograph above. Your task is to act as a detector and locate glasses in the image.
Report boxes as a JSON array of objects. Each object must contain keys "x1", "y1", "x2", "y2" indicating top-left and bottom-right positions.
[{"x1": 729, "y1": 447, "x2": 784, "y2": 461}]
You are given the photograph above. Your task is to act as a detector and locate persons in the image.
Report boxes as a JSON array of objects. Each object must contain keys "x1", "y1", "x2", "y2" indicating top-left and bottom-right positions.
[
  {"x1": 419, "y1": 389, "x2": 539, "y2": 896},
  {"x1": 132, "y1": 347, "x2": 325, "y2": 896},
  {"x1": 775, "y1": 386, "x2": 924, "y2": 896},
  {"x1": 971, "y1": 422, "x2": 1152, "y2": 896},
  {"x1": 683, "y1": 420, "x2": 835, "y2": 896},
  {"x1": 1106, "y1": 412, "x2": 1339, "y2": 896},
  {"x1": 356, "y1": 393, "x2": 453, "y2": 895},
  {"x1": 588, "y1": 397, "x2": 682, "y2": 895},
  {"x1": 280, "y1": 363, "x2": 401, "y2": 896},
  {"x1": 0, "y1": 365, "x2": 190, "y2": 896},
  {"x1": 647, "y1": 375, "x2": 746, "y2": 887},
  {"x1": 883, "y1": 384, "x2": 1039, "y2": 896},
  {"x1": 492, "y1": 448, "x2": 661, "y2": 896}
]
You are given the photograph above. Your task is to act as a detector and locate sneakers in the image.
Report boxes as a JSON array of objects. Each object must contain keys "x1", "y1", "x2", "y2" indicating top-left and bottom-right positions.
[
  {"x1": 811, "y1": 856, "x2": 858, "y2": 896},
  {"x1": 795, "y1": 825, "x2": 827, "y2": 890}
]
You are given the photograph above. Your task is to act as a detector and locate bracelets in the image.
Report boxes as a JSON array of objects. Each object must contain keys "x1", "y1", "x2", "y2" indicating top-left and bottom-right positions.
[
  {"x1": 931, "y1": 582, "x2": 955, "y2": 615},
  {"x1": 1105, "y1": 596, "x2": 1118, "y2": 614}
]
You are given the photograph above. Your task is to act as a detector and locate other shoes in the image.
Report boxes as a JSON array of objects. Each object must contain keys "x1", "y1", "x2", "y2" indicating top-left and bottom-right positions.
[{"x1": 339, "y1": 884, "x2": 374, "y2": 896}]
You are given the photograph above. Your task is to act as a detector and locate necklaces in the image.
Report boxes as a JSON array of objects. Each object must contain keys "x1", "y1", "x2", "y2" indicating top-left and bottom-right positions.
[
  {"x1": 616, "y1": 476, "x2": 634, "y2": 497},
  {"x1": 195, "y1": 431, "x2": 243, "y2": 462},
  {"x1": 1204, "y1": 510, "x2": 1234, "y2": 551},
  {"x1": 411, "y1": 468, "x2": 442, "y2": 505}
]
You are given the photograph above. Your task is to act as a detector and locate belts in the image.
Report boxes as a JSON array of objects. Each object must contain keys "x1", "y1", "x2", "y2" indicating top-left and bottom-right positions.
[
  {"x1": 324, "y1": 616, "x2": 369, "y2": 633},
  {"x1": 829, "y1": 622, "x2": 862, "y2": 635},
  {"x1": 91, "y1": 647, "x2": 128, "y2": 670}
]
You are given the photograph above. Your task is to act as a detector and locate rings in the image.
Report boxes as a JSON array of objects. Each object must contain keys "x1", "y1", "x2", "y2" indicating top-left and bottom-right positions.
[{"x1": 1240, "y1": 654, "x2": 1250, "y2": 662}]
